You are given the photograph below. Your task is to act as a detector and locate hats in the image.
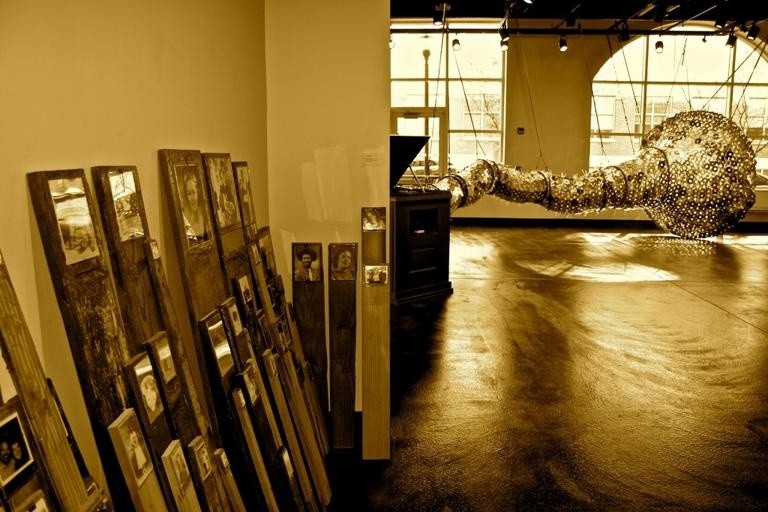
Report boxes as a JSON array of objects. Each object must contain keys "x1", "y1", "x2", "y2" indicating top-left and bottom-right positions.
[{"x1": 297, "y1": 249, "x2": 317, "y2": 261}]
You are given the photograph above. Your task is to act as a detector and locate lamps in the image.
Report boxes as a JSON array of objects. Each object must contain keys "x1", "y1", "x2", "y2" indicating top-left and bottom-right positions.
[
  {"x1": 713, "y1": 2, "x2": 761, "y2": 49},
  {"x1": 431, "y1": 9, "x2": 666, "y2": 57}
]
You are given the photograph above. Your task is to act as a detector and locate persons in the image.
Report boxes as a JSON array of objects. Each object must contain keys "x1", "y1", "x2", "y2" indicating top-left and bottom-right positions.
[
  {"x1": 0, "y1": 440, "x2": 16, "y2": 481},
  {"x1": 240, "y1": 169, "x2": 252, "y2": 224},
  {"x1": 176, "y1": 455, "x2": 188, "y2": 483},
  {"x1": 218, "y1": 160, "x2": 237, "y2": 225},
  {"x1": 367, "y1": 269, "x2": 374, "y2": 282},
  {"x1": 11, "y1": 440, "x2": 26, "y2": 470},
  {"x1": 378, "y1": 269, "x2": 386, "y2": 283},
  {"x1": 183, "y1": 171, "x2": 205, "y2": 238},
  {"x1": 332, "y1": 249, "x2": 353, "y2": 280},
  {"x1": 200, "y1": 450, "x2": 208, "y2": 474},
  {"x1": 295, "y1": 249, "x2": 320, "y2": 282},
  {"x1": 128, "y1": 423, "x2": 148, "y2": 475}
]
